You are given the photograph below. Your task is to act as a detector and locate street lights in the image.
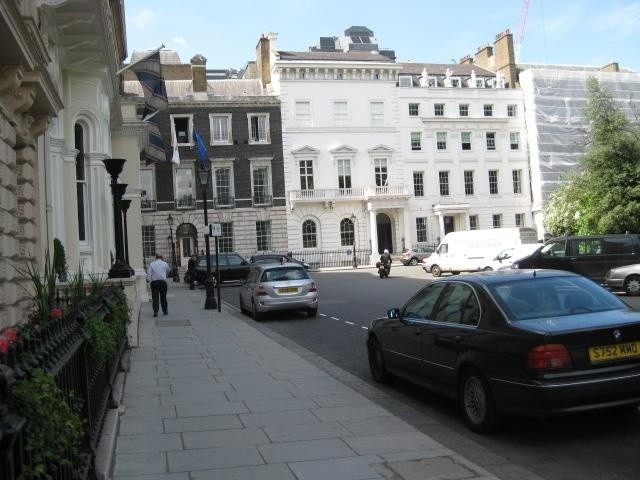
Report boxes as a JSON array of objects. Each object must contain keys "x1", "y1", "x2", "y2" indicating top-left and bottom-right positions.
[
  {"x1": 167, "y1": 214, "x2": 179, "y2": 282},
  {"x1": 192, "y1": 157, "x2": 218, "y2": 310}
]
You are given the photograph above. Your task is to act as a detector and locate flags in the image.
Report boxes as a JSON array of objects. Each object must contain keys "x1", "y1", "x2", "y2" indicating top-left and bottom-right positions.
[
  {"x1": 191, "y1": 128, "x2": 209, "y2": 163},
  {"x1": 129, "y1": 51, "x2": 180, "y2": 167}
]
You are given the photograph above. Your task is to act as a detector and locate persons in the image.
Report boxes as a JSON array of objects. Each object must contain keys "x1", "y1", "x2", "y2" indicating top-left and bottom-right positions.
[
  {"x1": 381, "y1": 249, "x2": 392, "y2": 274},
  {"x1": 188, "y1": 254, "x2": 197, "y2": 290},
  {"x1": 146, "y1": 254, "x2": 171, "y2": 317}
]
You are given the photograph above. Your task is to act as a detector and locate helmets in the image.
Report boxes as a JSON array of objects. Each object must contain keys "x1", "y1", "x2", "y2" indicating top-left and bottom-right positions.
[{"x1": 384, "y1": 248, "x2": 389, "y2": 255}]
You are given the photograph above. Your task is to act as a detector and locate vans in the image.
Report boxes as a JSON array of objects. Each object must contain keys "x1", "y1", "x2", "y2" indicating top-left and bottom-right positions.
[
  {"x1": 400, "y1": 246, "x2": 435, "y2": 266},
  {"x1": 423, "y1": 226, "x2": 538, "y2": 277},
  {"x1": 512, "y1": 235, "x2": 640, "y2": 275},
  {"x1": 194, "y1": 252, "x2": 250, "y2": 283}
]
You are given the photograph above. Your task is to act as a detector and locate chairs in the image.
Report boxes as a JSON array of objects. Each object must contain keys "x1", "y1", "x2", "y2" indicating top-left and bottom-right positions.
[
  {"x1": 564, "y1": 291, "x2": 595, "y2": 310},
  {"x1": 506, "y1": 286, "x2": 536, "y2": 312}
]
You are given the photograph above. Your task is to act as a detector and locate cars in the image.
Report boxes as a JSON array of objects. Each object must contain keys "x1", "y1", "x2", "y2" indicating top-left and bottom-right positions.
[
  {"x1": 602, "y1": 263, "x2": 639, "y2": 293},
  {"x1": 248, "y1": 254, "x2": 312, "y2": 272},
  {"x1": 366, "y1": 266, "x2": 639, "y2": 433},
  {"x1": 239, "y1": 261, "x2": 321, "y2": 318}
]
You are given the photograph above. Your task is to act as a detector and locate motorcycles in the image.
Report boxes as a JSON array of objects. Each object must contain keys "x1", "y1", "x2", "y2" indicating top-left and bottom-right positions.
[{"x1": 376, "y1": 260, "x2": 391, "y2": 277}]
